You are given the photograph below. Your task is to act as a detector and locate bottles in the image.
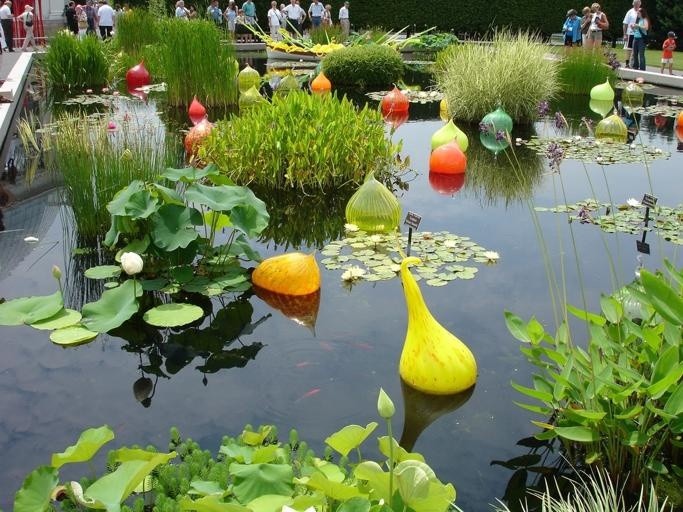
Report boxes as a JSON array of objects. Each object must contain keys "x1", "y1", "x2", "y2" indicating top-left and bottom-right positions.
[
  {"x1": 25, "y1": 5, "x2": 32, "y2": 12},
  {"x1": 667, "y1": 31, "x2": 676, "y2": 37}
]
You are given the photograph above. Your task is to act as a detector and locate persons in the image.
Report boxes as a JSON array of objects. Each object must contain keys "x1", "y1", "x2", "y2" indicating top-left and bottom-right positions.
[
  {"x1": 61, "y1": 0, "x2": 132, "y2": 42},
  {"x1": 622, "y1": 0, "x2": 642, "y2": 68},
  {"x1": 563, "y1": 9, "x2": 582, "y2": 48},
  {"x1": 17, "y1": 5, "x2": 39, "y2": 51},
  {"x1": 661, "y1": 31, "x2": 678, "y2": 75},
  {"x1": 581, "y1": 6, "x2": 594, "y2": 46},
  {"x1": 0, "y1": 0, "x2": 15, "y2": 51},
  {"x1": 590, "y1": 3, "x2": 609, "y2": 47},
  {"x1": 174, "y1": 1, "x2": 350, "y2": 43},
  {"x1": 631, "y1": 8, "x2": 650, "y2": 70}
]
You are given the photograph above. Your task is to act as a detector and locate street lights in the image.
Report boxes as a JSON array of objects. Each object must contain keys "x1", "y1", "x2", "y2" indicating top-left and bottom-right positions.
[
  {"x1": 78, "y1": 20, "x2": 86, "y2": 28},
  {"x1": 26, "y1": 22, "x2": 32, "y2": 26},
  {"x1": 641, "y1": 34, "x2": 650, "y2": 44}
]
